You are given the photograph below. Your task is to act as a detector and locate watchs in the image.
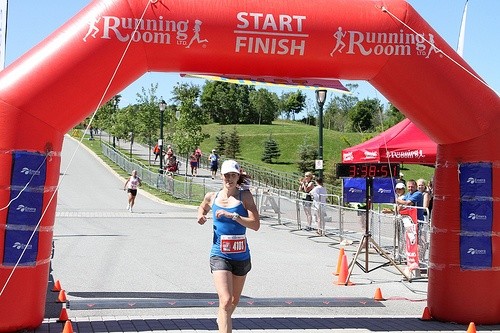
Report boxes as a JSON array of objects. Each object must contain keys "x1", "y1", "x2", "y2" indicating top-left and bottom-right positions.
[{"x1": 232, "y1": 212, "x2": 239, "y2": 220}]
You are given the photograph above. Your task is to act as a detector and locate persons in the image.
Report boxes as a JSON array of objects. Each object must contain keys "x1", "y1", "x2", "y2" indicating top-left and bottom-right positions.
[
  {"x1": 123, "y1": 170, "x2": 142, "y2": 213},
  {"x1": 190, "y1": 145, "x2": 202, "y2": 177},
  {"x1": 164, "y1": 145, "x2": 178, "y2": 176},
  {"x1": 298, "y1": 172, "x2": 314, "y2": 230},
  {"x1": 153, "y1": 144, "x2": 162, "y2": 163},
  {"x1": 382, "y1": 179, "x2": 432, "y2": 233},
  {"x1": 197, "y1": 159, "x2": 261, "y2": 333},
  {"x1": 309, "y1": 177, "x2": 329, "y2": 234},
  {"x1": 129, "y1": 132, "x2": 135, "y2": 145},
  {"x1": 208, "y1": 150, "x2": 220, "y2": 182}
]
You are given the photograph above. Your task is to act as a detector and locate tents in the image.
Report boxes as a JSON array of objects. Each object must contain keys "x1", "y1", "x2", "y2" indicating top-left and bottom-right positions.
[{"x1": 342, "y1": 117, "x2": 443, "y2": 164}]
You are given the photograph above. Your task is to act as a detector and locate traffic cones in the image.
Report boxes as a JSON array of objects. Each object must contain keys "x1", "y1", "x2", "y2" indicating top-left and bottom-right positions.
[
  {"x1": 465, "y1": 321, "x2": 478, "y2": 333},
  {"x1": 372, "y1": 287, "x2": 386, "y2": 301},
  {"x1": 333, "y1": 255, "x2": 354, "y2": 285},
  {"x1": 418, "y1": 307, "x2": 434, "y2": 321},
  {"x1": 333, "y1": 248, "x2": 346, "y2": 276}
]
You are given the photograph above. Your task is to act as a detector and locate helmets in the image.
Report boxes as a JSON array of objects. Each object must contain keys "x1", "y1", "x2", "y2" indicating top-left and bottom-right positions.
[{"x1": 305, "y1": 172, "x2": 313, "y2": 176}]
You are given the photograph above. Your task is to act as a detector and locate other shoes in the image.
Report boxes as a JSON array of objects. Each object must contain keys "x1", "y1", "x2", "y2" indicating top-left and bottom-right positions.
[
  {"x1": 130, "y1": 209, "x2": 133, "y2": 213},
  {"x1": 212, "y1": 178, "x2": 215, "y2": 180},
  {"x1": 128, "y1": 206, "x2": 130, "y2": 210}
]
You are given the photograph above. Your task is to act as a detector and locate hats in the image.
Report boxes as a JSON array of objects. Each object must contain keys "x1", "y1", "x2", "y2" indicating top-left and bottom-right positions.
[
  {"x1": 167, "y1": 151, "x2": 172, "y2": 155},
  {"x1": 212, "y1": 149, "x2": 217, "y2": 152},
  {"x1": 399, "y1": 173, "x2": 404, "y2": 176},
  {"x1": 221, "y1": 160, "x2": 240, "y2": 174},
  {"x1": 167, "y1": 145, "x2": 171, "y2": 148},
  {"x1": 396, "y1": 183, "x2": 406, "y2": 189}
]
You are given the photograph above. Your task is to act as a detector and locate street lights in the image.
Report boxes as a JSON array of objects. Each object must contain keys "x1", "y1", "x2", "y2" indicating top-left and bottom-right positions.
[
  {"x1": 314, "y1": 88, "x2": 327, "y2": 185},
  {"x1": 158, "y1": 99, "x2": 167, "y2": 174}
]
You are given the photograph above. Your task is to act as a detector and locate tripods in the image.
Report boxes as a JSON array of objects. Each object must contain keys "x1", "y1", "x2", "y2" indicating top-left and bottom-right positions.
[{"x1": 344, "y1": 178, "x2": 410, "y2": 286}]
